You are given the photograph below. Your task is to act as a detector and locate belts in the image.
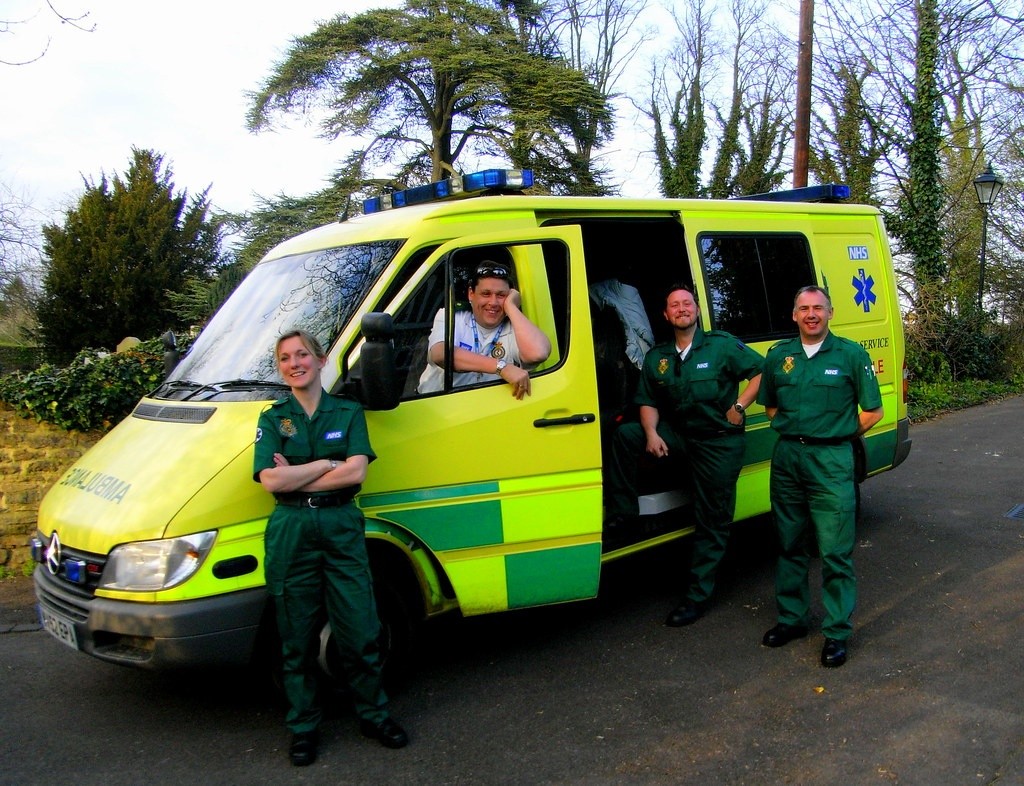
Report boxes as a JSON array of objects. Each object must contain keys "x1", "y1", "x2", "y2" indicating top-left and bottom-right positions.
[
  {"x1": 275, "y1": 493, "x2": 355, "y2": 508},
  {"x1": 780, "y1": 433, "x2": 851, "y2": 446}
]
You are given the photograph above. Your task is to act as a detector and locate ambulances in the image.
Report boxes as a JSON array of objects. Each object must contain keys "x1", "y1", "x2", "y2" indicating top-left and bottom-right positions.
[{"x1": 31, "y1": 168, "x2": 912, "y2": 713}]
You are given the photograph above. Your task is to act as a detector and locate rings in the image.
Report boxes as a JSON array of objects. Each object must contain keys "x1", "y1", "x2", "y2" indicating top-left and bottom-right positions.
[{"x1": 520, "y1": 385, "x2": 524, "y2": 387}]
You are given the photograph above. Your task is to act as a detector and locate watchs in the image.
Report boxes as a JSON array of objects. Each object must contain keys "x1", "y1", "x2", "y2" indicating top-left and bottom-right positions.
[
  {"x1": 496, "y1": 359, "x2": 507, "y2": 376},
  {"x1": 734, "y1": 400, "x2": 744, "y2": 414}
]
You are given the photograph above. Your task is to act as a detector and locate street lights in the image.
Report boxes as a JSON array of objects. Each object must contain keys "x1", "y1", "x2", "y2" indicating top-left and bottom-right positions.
[{"x1": 973, "y1": 161, "x2": 1004, "y2": 328}]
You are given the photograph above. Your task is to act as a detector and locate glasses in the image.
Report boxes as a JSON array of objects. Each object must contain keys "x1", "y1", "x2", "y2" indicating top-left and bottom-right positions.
[
  {"x1": 674, "y1": 351, "x2": 684, "y2": 377},
  {"x1": 470, "y1": 266, "x2": 508, "y2": 281}
]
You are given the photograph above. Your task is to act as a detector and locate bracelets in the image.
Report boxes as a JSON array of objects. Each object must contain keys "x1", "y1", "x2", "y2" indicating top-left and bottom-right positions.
[{"x1": 330, "y1": 459, "x2": 337, "y2": 470}]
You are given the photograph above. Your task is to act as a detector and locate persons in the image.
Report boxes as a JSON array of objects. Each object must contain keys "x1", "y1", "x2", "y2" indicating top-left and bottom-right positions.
[
  {"x1": 757, "y1": 286, "x2": 885, "y2": 668},
  {"x1": 415, "y1": 260, "x2": 551, "y2": 401},
  {"x1": 604, "y1": 287, "x2": 767, "y2": 627},
  {"x1": 252, "y1": 331, "x2": 412, "y2": 767}
]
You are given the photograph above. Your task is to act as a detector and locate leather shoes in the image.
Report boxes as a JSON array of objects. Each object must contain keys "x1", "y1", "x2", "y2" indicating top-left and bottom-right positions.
[
  {"x1": 822, "y1": 637, "x2": 848, "y2": 666},
  {"x1": 604, "y1": 512, "x2": 637, "y2": 529},
  {"x1": 763, "y1": 622, "x2": 809, "y2": 648},
  {"x1": 665, "y1": 600, "x2": 704, "y2": 627},
  {"x1": 359, "y1": 715, "x2": 408, "y2": 749},
  {"x1": 289, "y1": 732, "x2": 320, "y2": 766}
]
